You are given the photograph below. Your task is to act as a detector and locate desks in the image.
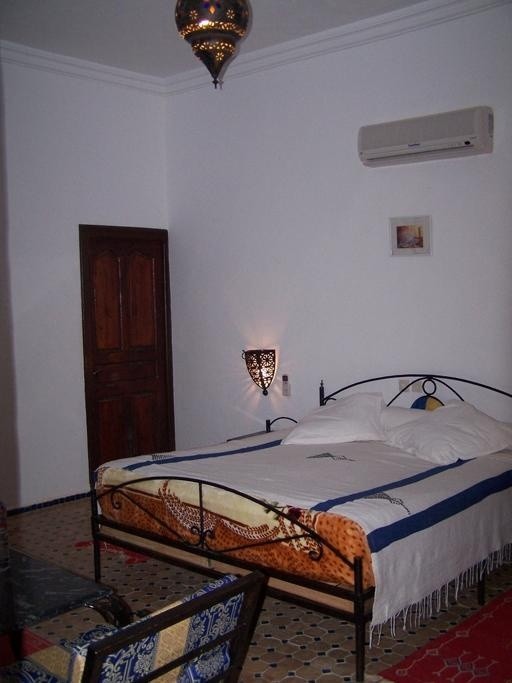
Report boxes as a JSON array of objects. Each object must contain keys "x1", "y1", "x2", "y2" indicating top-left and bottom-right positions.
[{"x1": 1, "y1": 546, "x2": 113, "y2": 657}]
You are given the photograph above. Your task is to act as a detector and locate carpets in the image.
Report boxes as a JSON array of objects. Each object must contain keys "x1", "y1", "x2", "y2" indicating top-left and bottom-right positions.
[{"x1": 378, "y1": 583, "x2": 512, "y2": 681}]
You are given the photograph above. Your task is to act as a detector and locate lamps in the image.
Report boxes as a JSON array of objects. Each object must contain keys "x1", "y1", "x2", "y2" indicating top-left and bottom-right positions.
[
  {"x1": 173, "y1": 2, "x2": 250, "y2": 91},
  {"x1": 241, "y1": 346, "x2": 279, "y2": 396}
]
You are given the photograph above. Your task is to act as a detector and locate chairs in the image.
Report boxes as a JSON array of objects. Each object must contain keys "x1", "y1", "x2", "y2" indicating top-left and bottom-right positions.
[{"x1": 2, "y1": 569, "x2": 270, "y2": 683}]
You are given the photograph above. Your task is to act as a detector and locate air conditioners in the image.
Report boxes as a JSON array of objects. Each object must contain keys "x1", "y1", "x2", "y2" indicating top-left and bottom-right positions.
[{"x1": 357, "y1": 107, "x2": 496, "y2": 166}]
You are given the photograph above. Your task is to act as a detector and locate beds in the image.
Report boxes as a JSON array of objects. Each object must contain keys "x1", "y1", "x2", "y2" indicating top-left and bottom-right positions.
[{"x1": 88, "y1": 373, "x2": 511, "y2": 681}]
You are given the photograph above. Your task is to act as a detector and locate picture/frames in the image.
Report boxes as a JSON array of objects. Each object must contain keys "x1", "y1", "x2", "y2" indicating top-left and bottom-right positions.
[{"x1": 389, "y1": 215, "x2": 431, "y2": 255}]
